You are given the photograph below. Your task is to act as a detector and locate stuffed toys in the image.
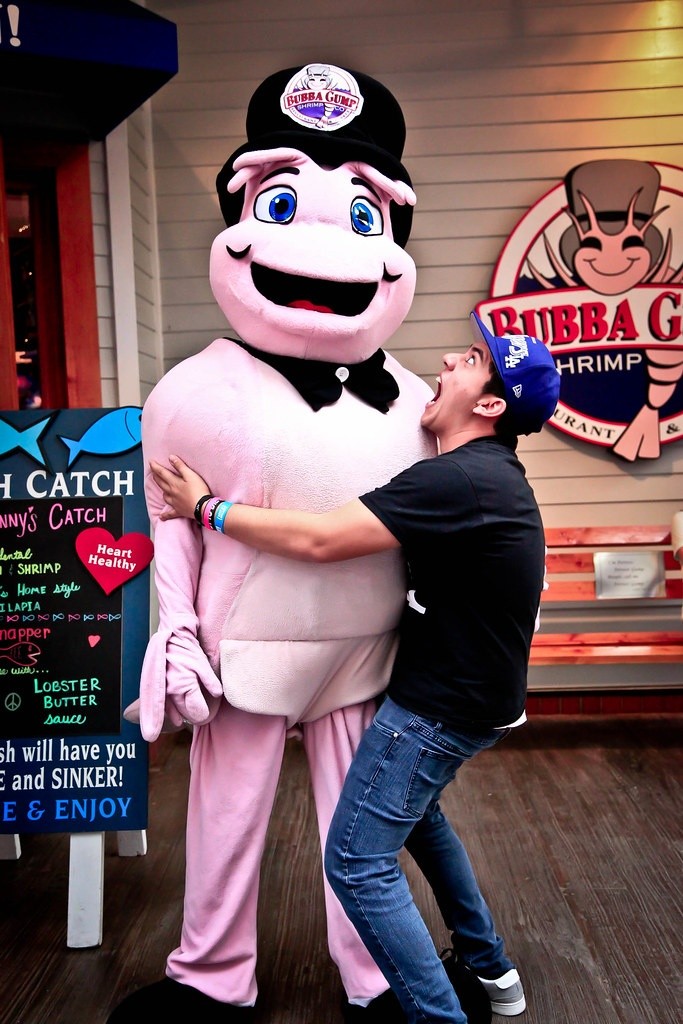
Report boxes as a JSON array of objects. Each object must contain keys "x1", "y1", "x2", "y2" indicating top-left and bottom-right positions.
[{"x1": 106, "y1": 62, "x2": 439, "y2": 1024}]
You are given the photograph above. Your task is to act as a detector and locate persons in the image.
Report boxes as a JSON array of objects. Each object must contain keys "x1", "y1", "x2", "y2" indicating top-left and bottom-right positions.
[{"x1": 150, "y1": 311, "x2": 559, "y2": 1024}]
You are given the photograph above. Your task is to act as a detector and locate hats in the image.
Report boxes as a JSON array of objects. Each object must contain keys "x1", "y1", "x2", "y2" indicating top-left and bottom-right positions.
[{"x1": 468, "y1": 310, "x2": 560, "y2": 433}]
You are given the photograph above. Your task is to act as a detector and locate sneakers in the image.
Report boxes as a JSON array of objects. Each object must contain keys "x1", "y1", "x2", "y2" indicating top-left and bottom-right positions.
[{"x1": 439, "y1": 948, "x2": 526, "y2": 1017}]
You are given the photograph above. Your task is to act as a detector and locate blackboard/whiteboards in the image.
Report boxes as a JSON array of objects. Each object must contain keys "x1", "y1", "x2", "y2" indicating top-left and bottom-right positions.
[{"x1": 0, "y1": 497, "x2": 123, "y2": 740}]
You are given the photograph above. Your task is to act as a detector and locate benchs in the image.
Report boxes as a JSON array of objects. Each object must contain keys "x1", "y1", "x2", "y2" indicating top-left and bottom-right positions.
[{"x1": 527, "y1": 526, "x2": 683, "y2": 664}]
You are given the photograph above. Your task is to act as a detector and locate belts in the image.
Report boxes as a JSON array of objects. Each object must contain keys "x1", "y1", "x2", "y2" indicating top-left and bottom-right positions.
[{"x1": 422, "y1": 712, "x2": 511, "y2": 740}]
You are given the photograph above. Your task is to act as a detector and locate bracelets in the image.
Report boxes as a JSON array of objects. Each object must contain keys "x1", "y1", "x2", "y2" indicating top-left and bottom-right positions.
[{"x1": 195, "y1": 495, "x2": 233, "y2": 534}]
